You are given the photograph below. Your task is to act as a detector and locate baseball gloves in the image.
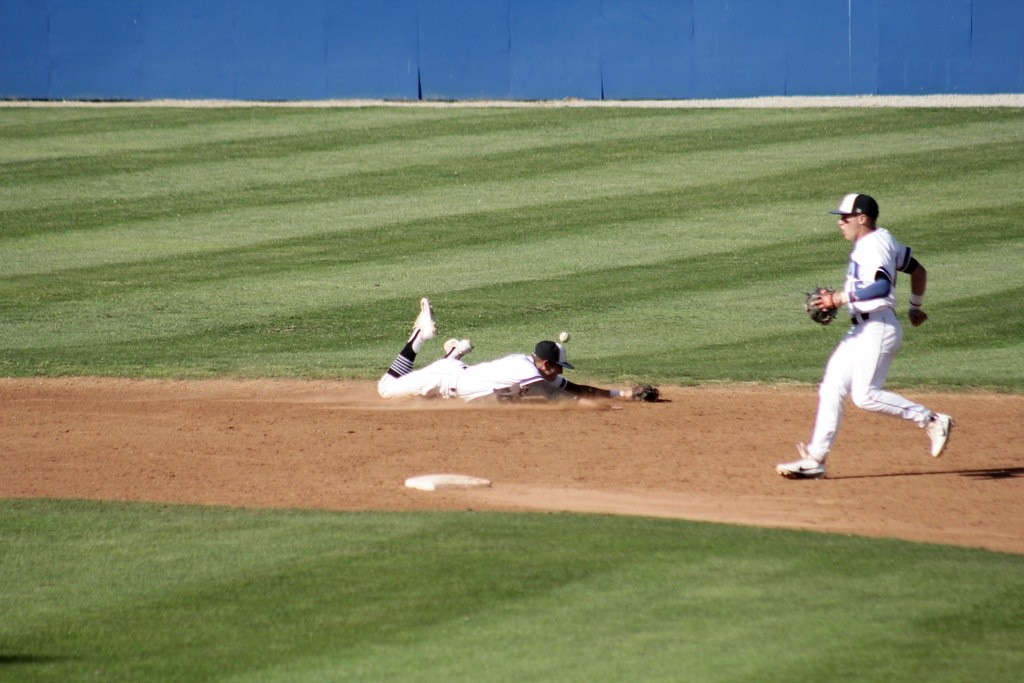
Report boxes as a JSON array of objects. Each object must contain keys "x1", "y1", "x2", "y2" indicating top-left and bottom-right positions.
[
  {"x1": 805, "y1": 288, "x2": 838, "y2": 325},
  {"x1": 632, "y1": 383, "x2": 659, "y2": 402}
]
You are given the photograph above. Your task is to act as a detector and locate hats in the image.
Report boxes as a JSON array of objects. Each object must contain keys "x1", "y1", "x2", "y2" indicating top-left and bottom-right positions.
[
  {"x1": 535, "y1": 340, "x2": 575, "y2": 371},
  {"x1": 828, "y1": 193, "x2": 878, "y2": 217}
]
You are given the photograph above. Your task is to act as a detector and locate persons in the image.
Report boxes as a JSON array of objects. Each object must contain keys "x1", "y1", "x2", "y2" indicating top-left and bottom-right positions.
[
  {"x1": 776, "y1": 192, "x2": 956, "y2": 478},
  {"x1": 377, "y1": 296, "x2": 659, "y2": 412}
]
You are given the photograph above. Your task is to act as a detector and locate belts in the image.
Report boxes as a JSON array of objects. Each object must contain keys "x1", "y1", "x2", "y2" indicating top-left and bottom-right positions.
[
  {"x1": 451, "y1": 366, "x2": 467, "y2": 392},
  {"x1": 850, "y1": 313, "x2": 870, "y2": 325}
]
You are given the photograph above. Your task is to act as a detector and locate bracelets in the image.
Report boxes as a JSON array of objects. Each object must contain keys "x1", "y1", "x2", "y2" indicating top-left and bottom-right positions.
[
  {"x1": 841, "y1": 291, "x2": 857, "y2": 304},
  {"x1": 609, "y1": 390, "x2": 626, "y2": 398},
  {"x1": 909, "y1": 294, "x2": 924, "y2": 310}
]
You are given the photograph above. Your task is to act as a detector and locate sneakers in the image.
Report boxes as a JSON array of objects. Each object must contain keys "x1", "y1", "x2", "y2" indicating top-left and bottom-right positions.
[
  {"x1": 776, "y1": 442, "x2": 825, "y2": 478},
  {"x1": 444, "y1": 338, "x2": 475, "y2": 357},
  {"x1": 412, "y1": 297, "x2": 438, "y2": 341},
  {"x1": 925, "y1": 413, "x2": 954, "y2": 457}
]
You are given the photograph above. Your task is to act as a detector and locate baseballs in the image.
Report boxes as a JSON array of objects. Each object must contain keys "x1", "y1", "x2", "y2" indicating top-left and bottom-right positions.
[{"x1": 560, "y1": 332, "x2": 570, "y2": 343}]
